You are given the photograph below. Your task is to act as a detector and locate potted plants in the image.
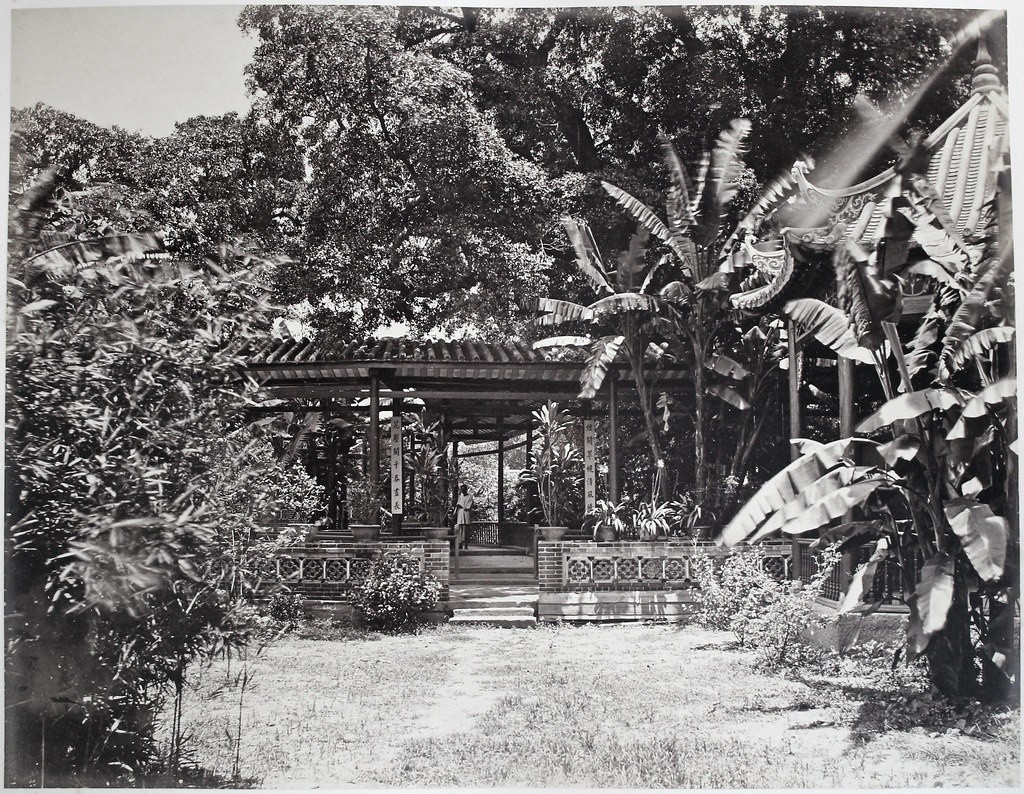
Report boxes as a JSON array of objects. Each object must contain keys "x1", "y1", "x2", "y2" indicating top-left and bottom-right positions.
[
  {"x1": 278, "y1": 457, "x2": 331, "y2": 541},
  {"x1": 518, "y1": 399, "x2": 584, "y2": 541},
  {"x1": 670, "y1": 492, "x2": 716, "y2": 539},
  {"x1": 337, "y1": 472, "x2": 390, "y2": 543},
  {"x1": 401, "y1": 407, "x2": 465, "y2": 543}
]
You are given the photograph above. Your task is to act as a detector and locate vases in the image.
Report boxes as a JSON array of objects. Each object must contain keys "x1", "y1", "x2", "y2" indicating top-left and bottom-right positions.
[
  {"x1": 597, "y1": 526, "x2": 616, "y2": 542},
  {"x1": 636, "y1": 525, "x2": 661, "y2": 541}
]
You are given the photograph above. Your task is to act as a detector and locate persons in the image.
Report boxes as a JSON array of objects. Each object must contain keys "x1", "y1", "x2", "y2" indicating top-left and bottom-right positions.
[{"x1": 453, "y1": 485, "x2": 474, "y2": 549}]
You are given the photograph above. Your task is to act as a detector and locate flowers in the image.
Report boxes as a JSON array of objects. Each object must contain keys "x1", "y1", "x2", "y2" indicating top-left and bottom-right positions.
[
  {"x1": 632, "y1": 459, "x2": 676, "y2": 541},
  {"x1": 580, "y1": 462, "x2": 628, "y2": 540}
]
